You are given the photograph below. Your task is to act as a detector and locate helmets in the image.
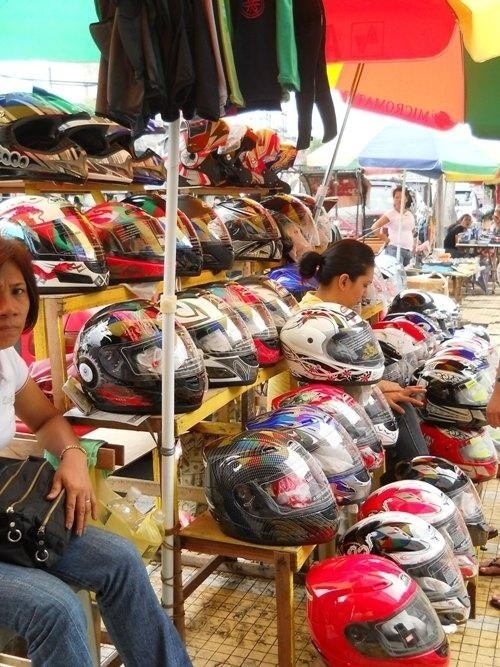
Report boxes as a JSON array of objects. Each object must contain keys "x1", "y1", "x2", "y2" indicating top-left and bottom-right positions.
[{"x1": 0, "y1": 93, "x2": 498, "y2": 667}]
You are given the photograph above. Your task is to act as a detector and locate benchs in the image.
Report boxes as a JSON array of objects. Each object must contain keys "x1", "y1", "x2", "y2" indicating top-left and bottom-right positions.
[{"x1": 178, "y1": 416, "x2": 385, "y2": 667}]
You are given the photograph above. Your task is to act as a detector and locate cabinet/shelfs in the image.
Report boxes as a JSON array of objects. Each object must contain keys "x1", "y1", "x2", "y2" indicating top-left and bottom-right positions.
[{"x1": 0, "y1": 175, "x2": 384, "y2": 506}]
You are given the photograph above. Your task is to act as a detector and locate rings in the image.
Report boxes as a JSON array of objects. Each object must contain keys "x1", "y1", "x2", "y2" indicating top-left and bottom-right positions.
[{"x1": 86, "y1": 499, "x2": 90, "y2": 503}]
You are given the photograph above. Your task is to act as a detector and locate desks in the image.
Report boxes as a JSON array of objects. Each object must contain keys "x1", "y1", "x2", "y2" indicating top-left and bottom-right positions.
[{"x1": 455, "y1": 242, "x2": 500, "y2": 296}]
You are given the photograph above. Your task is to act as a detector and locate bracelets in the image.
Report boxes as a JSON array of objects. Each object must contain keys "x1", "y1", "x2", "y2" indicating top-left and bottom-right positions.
[
  {"x1": 495, "y1": 376, "x2": 500, "y2": 382},
  {"x1": 59, "y1": 445, "x2": 87, "y2": 461}
]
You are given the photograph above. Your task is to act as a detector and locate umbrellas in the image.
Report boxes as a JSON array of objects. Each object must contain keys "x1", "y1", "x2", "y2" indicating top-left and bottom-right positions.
[
  {"x1": 314, "y1": 1, "x2": 499, "y2": 224},
  {"x1": 354, "y1": 126, "x2": 500, "y2": 264},
  {"x1": 0, "y1": 1, "x2": 499, "y2": 630}
]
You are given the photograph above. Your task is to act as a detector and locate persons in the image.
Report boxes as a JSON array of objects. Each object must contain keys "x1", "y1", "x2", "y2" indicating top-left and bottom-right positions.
[
  {"x1": 370, "y1": 186, "x2": 418, "y2": 267},
  {"x1": 474, "y1": 214, "x2": 494, "y2": 239},
  {"x1": 443, "y1": 214, "x2": 474, "y2": 257},
  {"x1": 1, "y1": 235, "x2": 194, "y2": 667},
  {"x1": 479, "y1": 557, "x2": 500, "y2": 611},
  {"x1": 292, "y1": 238, "x2": 498, "y2": 541}
]
